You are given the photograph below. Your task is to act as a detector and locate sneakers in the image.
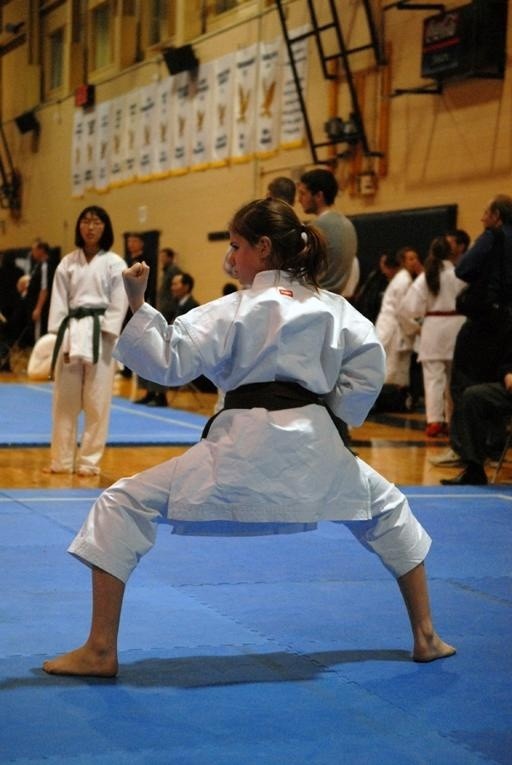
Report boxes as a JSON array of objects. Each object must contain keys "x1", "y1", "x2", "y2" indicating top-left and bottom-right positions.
[
  {"x1": 429, "y1": 450, "x2": 461, "y2": 469},
  {"x1": 425, "y1": 422, "x2": 442, "y2": 436}
]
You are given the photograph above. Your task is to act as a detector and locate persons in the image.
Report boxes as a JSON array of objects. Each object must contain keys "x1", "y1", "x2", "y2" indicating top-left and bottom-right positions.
[
  {"x1": 39, "y1": 196, "x2": 461, "y2": 682},
  {"x1": 425, "y1": 185, "x2": 512, "y2": 469},
  {"x1": 0, "y1": 166, "x2": 473, "y2": 437},
  {"x1": 39, "y1": 204, "x2": 132, "y2": 480},
  {"x1": 436, "y1": 371, "x2": 512, "y2": 485}
]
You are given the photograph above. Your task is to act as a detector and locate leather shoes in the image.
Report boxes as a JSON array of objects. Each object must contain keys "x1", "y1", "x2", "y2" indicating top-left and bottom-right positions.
[
  {"x1": 155, "y1": 393, "x2": 168, "y2": 406},
  {"x1": 136, "y1": 391, "x2": 155, "y2": 404},
  {"x1": 440, "y1": 469, "x2": 490, "y2": 485}
]
